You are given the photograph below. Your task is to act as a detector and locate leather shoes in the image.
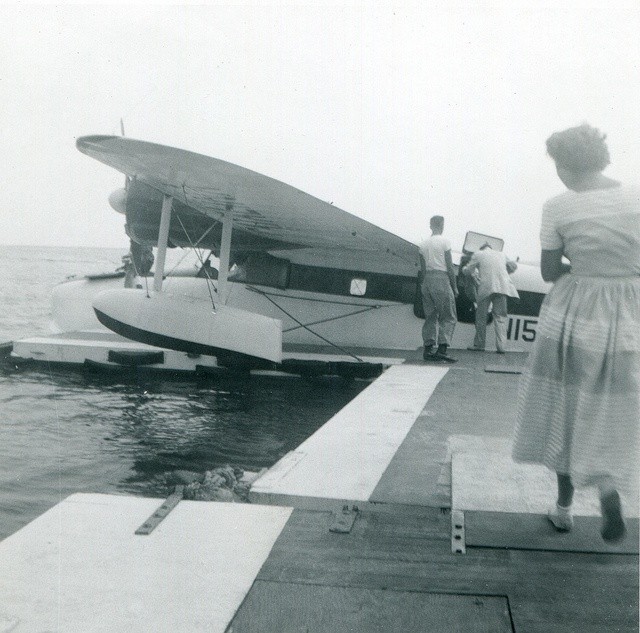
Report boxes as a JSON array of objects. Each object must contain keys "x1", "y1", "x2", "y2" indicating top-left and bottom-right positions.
[
  {"x1": 467, "y1": 344, "x2": 484, "y2": 351},
  {"x1": 548, "y1": 507, "x2": 574, "y2": 529},
  {"x1": 601, "y1": 490, "x2": 625, "y2": 545}
]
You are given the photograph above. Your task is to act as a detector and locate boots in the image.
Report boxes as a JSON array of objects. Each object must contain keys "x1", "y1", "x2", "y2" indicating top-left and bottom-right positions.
[
  {"x1": 435, "y1": 344, "x2": 458, "y2": 362},
  {"x1": 423, "y1": 344, "x2": 437, "y2": 361}
]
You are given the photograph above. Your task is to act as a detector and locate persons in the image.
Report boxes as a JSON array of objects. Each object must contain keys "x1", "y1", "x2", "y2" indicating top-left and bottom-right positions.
[
  {"x1": 512, "y1": 121, "x2": 639, "y2": 539},
  {"x1": 419, "y1": 215, "x2": 457, "y2": 363},
  {"x1": 461, "y1": 243, "x2": 522, "y2": 354}
]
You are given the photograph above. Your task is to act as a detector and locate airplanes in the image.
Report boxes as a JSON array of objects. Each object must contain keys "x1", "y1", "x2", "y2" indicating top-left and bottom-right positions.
[{"x1": 9, "y1": 134, "x2": 557, "y2": 380}]
